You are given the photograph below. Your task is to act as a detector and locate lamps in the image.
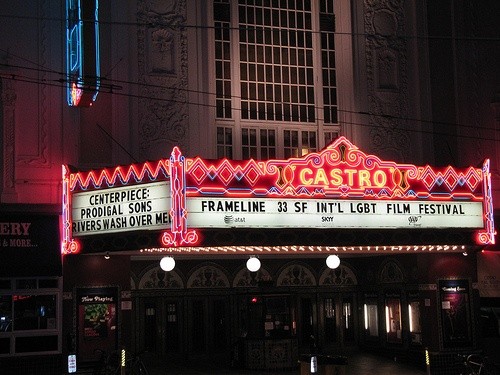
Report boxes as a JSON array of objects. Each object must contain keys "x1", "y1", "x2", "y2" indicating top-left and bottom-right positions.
[
  {"x1": 160, "y1": 255, "x2": 175, "y2": 271},
  {"x1": 246, "y1": 255, "x2": 262, "y2": 272},
  {"x1": 104, "y1": 251, "x2": 110, "y2": 259},
  {"x1": 325, "y1": 254, "x2": 340, "y2": 269}
]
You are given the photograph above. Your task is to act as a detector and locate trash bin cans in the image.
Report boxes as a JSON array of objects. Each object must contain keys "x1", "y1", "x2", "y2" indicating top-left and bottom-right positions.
[{"x1": 299, "y1": 354, "x2": 349, "y2": 375}]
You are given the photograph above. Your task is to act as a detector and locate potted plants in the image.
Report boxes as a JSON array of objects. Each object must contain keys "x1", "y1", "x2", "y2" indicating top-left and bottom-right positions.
[{"x1": 299, "y1": 335, "x2": 350, "y2": 375}]
